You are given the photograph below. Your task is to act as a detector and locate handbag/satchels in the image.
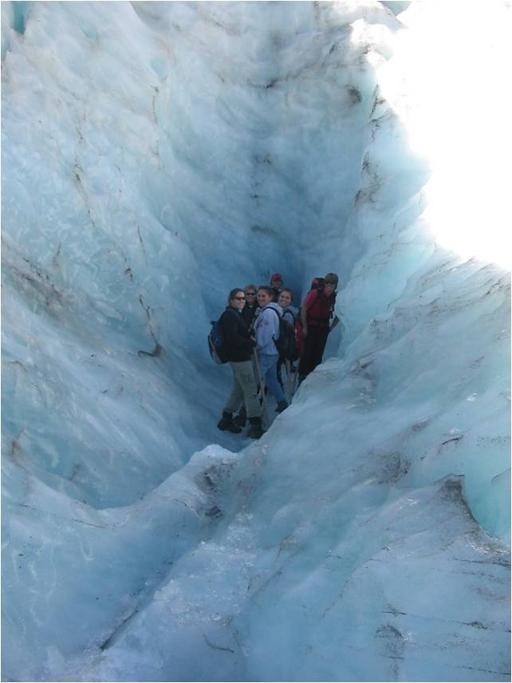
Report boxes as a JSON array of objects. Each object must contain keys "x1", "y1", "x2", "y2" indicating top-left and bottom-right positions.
[{"x1": 283, "y1": 308, "x2": 302, "y2": 352}]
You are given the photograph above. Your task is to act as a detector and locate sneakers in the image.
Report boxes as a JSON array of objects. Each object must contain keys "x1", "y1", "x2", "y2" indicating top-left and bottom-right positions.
[
  {"x1": 217, "y1": 410, "x2": 265, "y2": 438},
  {"x1": 276, "y1": 400, "x2": 288, "y2": 412}
]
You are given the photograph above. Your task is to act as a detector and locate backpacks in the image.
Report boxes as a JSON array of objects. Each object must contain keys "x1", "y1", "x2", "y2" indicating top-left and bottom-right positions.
[
  {"x1": 311, "y1": 278, "x2": 325, "y2": 304},
  {"x1": 263, "y1": 307, "x2": 297, "y2": 363},
  {"x1": 208, "y1": 309, "x2": 241, "y2": 364}
]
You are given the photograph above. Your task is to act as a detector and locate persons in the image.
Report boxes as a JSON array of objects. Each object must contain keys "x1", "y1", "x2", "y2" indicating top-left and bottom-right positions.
[
  {"x1": 233, "y1": 272, "x2": 340, "y2": 428},
  {"x1": 217, "y1": 288, "x2": 267, "y2": 440}
]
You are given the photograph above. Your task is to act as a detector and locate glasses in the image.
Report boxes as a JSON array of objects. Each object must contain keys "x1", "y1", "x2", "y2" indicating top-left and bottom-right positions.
[{"x1": 235, "y1": 296, "x2": 245, "y2": 300}]
